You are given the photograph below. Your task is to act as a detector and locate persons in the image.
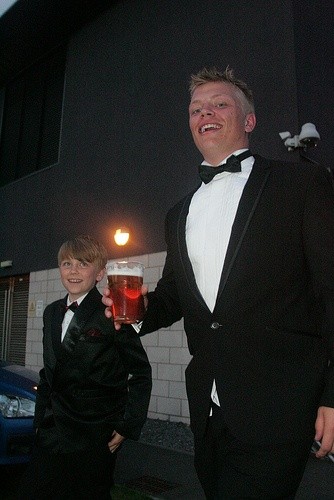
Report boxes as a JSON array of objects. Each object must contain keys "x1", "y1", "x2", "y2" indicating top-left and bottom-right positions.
[
  {"x1": 28, "y1": 235, "x2": 152, "y2": 500},
  {"x1": 101, "y1": 63, "x2": 334, "y2": 500}
]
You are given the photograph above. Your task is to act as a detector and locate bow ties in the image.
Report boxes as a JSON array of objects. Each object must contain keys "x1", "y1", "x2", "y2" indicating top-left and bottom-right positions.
[
  {"x1": 61, "y1": 301, "x2": 79, "y2": 314},
  {"x1": 199, "y1": 150, "x2": 253, "y2": 185}
]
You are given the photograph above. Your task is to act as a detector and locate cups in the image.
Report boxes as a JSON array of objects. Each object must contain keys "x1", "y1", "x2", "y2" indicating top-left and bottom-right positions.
[{"x1": 107, "y1": 260, "x2": 144, "y2": 326}]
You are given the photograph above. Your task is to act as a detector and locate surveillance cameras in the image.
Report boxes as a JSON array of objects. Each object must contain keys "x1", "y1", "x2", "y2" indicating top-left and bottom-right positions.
[{"x1": 299, "y1": 123, "x2": 321, "y2": 146}]
